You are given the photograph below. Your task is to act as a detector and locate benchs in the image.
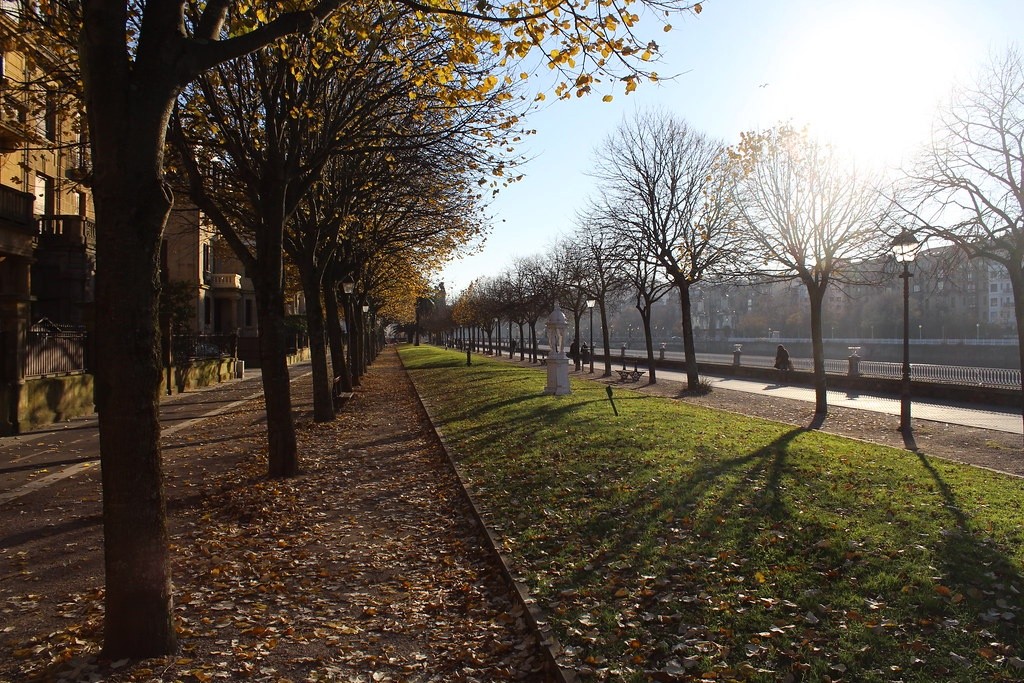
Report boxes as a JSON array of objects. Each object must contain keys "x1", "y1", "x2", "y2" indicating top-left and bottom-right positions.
[
  {"x1": 537, "y1": 358, "x2": 546, "y2": 364},
  {"x1": 616, "y1": 370, "x2": 646, "y2": 383}
]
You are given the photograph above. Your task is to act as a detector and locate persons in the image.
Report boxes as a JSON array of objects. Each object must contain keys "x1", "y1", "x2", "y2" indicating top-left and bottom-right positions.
[
  {"x1": 512, "y1": 338, "x2": 517, "y2": 355},
  {"x1": 570, "y1": 339, "x2": 576, "y2": 364},
  {"x1": 774, "y1": 344, "x2": 789, "y2": 387},
  {"x1": 580, "y1": 342, "x2": 589, "y2": 364}
]
